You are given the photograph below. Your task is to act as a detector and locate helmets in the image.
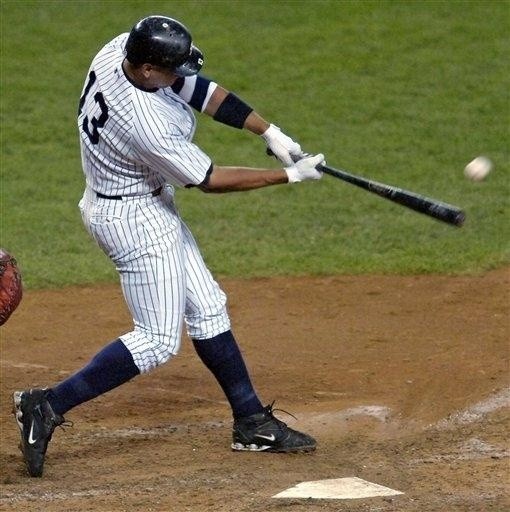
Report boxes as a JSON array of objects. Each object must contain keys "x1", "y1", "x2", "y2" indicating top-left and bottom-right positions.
[{"x1": 124, "y1": 15, "x2": 204, "y2": 76}]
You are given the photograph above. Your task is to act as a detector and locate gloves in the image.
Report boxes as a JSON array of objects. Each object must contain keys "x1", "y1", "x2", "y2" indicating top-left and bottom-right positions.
[{"x1": 261, "y1": 123, "x2": 327, "y2": 184}]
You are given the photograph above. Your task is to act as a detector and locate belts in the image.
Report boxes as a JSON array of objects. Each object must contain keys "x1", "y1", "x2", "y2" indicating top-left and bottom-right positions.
[{"x1": 93, "y1": 187, "x2": 163, "y2": 201}]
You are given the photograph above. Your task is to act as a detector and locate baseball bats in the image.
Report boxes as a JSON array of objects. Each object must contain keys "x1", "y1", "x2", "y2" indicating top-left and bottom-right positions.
[{"x1": 266, "y1": 147, "x2": 465, "y2": 227}]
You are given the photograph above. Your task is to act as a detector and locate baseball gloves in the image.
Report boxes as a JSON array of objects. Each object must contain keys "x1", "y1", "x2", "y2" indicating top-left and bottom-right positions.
[{"x1": 0, "y1": 248, "x2": 22, "y2": 326}]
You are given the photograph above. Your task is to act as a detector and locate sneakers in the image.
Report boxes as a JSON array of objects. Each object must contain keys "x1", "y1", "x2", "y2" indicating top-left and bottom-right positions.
[
  {"x1": 230, "y1": 400, "x2": 318, "y2": 453},
  {"x1": 13, "y1": 387, "x2": 74, "y2": 478}
]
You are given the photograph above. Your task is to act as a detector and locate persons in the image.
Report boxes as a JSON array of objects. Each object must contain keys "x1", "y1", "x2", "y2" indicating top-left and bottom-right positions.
[{"x1": 12, "y1": 15, "x2": 327, "y2": 478}]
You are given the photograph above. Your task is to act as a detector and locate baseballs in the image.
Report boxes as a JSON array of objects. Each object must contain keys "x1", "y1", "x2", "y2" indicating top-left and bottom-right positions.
[{"x1": 465, "y1": 157, "x2": 492, "y2": 179}]
